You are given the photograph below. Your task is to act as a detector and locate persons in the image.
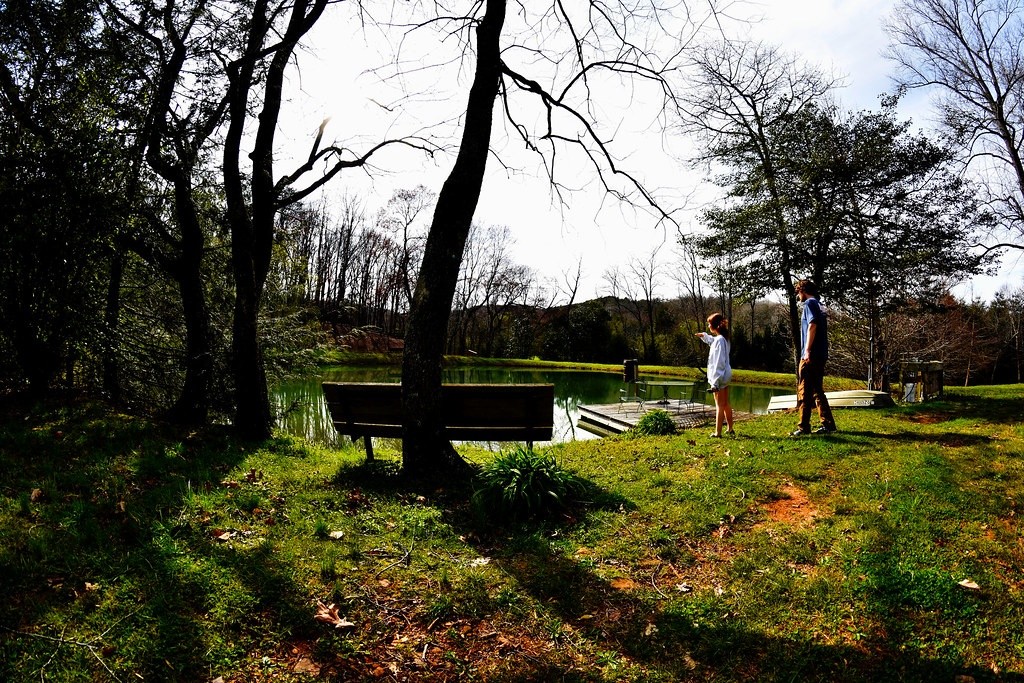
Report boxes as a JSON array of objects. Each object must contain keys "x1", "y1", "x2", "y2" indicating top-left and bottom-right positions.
[
  {"x1": 694, "y1": 312, "x2": 735, "y2": 437},
  {"x1": 789, "y1": 280, "x2": 837, "y2": 438}
]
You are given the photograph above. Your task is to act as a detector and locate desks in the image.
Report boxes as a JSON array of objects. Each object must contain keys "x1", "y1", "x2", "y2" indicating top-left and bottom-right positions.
[{"x1": 634, "y1": 380, "x2": 694, "y2": 405}]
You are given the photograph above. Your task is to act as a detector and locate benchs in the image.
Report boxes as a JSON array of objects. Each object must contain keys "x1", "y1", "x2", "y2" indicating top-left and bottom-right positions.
[{"x1": 322, "y1": 381, "x2": 555, "y2": 460}]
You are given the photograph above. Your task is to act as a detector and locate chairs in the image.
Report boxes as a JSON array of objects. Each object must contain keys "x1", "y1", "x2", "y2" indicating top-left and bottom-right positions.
[
  {"x1": 619, "y1": 389, "x2": 648, "y2": 418},
  {"x1": 678, "y1": 391, "x2": 704, "y2": 411}
]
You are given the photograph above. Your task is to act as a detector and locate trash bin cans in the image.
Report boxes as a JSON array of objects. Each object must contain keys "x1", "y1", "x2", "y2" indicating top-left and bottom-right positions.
[{"x1": 901, "y1": 361, "x2": 944, "y2": 404}]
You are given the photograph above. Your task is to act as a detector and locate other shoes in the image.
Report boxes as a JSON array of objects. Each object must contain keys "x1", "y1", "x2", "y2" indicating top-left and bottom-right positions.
[
  {"x1": 790, "y1": 429, "x2": 812, "y2": 436},
  {"x1": 710, "y1": 434, "x2": 723, "y2": 438},
  {"x1": 814, "y1": 426, "x2": 837, "y2": 434},
  {"x1": 724, "y1": 429, "x2": 734, "y2": 434}
]
